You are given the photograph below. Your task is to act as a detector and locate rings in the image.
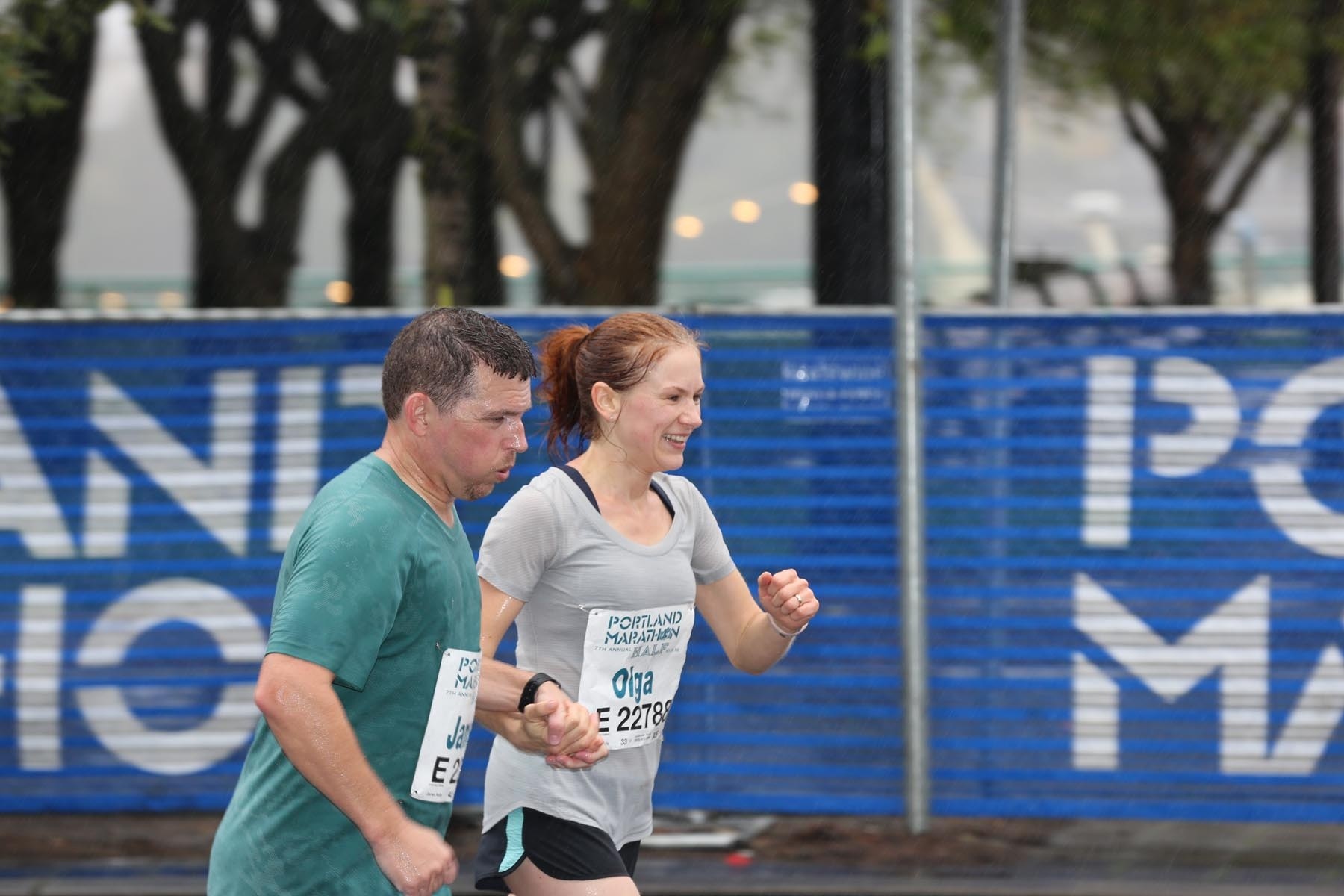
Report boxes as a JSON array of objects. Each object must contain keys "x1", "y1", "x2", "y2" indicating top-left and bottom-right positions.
[{"x1": 794, "y1": 595, "x2": 803, "y2": 606}]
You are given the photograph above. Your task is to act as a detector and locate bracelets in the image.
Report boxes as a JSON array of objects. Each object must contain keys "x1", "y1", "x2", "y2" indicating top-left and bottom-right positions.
[
  {"x1": 768, "y1": 610, "x2": 808, "y2": 636},
  {"x1": 519, "y1": 672, "x2": 561, "y2": 714}
]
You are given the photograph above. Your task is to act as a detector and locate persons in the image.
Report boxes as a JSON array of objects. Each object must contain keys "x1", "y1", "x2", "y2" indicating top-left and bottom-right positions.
[
  {"x1": 207, "y1": 303, "x2": 612, "y2": 896},
  {"x1": 472, "y1": 316, "x2": 821, "y2": 896}
]
